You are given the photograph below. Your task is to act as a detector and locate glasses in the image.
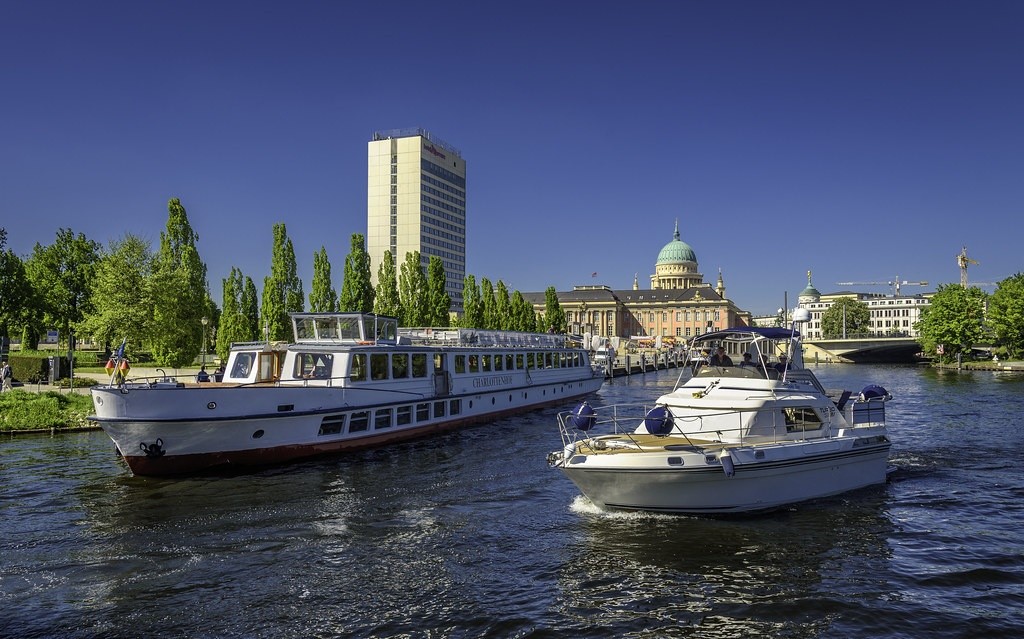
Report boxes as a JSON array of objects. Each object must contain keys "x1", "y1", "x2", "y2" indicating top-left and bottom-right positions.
[{"x1": 779, "y1": 358, "x2": 785, "y2": 359}]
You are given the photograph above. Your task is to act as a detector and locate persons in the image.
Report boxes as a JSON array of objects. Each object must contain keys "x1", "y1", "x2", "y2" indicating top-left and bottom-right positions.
[
  {"x1": 774, "y1": 354, "x2": 791, "y2": 374},
  {"x1": 710, "y1": 347, "x2": 734, "y2": 366},
  {"x1": 757, "y1": 354, "x2": 772, "y2": 367},
  {"x1": 0, "y1": 361, "x2": 13, "y2": 392},
  {"x1": 739, "y1": 352, "x2": 756, "y2": 368},
  {"x1": 196, "y1": 366, "x2": 224, "y2": 383}
]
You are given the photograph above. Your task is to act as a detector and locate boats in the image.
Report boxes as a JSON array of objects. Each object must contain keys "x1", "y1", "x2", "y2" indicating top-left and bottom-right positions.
[
  {"x1": 545, "y1": 291, "x2": 892, "y2": 514},
  {"x1": 86, "y1": 311, "x2": 604, "y2": 479}
]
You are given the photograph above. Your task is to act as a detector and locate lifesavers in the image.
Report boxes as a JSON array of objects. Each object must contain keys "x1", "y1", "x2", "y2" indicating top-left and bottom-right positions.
[{"x1": 358, "y1": 340, "x2": 376, "y2": 345}]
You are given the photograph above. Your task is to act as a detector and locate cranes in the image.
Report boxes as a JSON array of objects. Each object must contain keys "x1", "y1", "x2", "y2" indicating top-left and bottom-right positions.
[
  {"x1": 956, "y1": 246, "x2": 979, "y2": 287},
  {"x1": 838, "y1": 276, "x2": 929, "y2": 295}
]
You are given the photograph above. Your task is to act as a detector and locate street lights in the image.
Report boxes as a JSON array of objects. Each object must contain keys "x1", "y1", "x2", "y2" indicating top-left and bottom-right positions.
[{"x1": 201, "y1": 315, "x2": 208, "y2": 367}]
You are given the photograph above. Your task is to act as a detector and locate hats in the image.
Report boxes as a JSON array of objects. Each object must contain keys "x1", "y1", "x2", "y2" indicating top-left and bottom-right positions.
[
  {"x1": 780, "y1": 355, "x2": 787, "y2": 361},
  {"x1": 743, "y1": 352, "x2": 752, "y2": 359}
]
